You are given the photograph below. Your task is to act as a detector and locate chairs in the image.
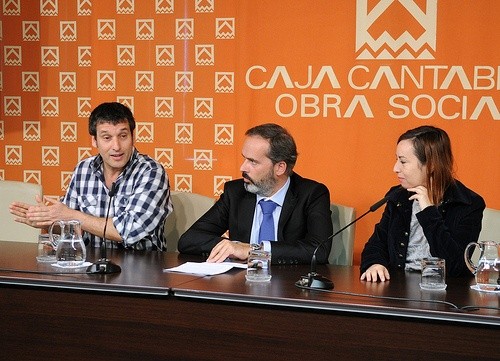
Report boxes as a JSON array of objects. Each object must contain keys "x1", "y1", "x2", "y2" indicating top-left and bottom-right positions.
[
  {"x1": 469, "y1": 209, "x2": 500, "y2": 264},
  {"x1": 162, "y1": 191, "x2": 216, "y2": 252},
  {"x1": 0, "y1": 181, "x2": 42, "y2": 244},
  {"x1": 329, "y1": 204, "x2": 356, "y2": 267}
]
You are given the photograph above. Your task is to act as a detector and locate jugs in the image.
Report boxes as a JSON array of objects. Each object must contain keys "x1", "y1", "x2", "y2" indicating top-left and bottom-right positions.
[{"x1": 463, "y1": 240, "x2": 500, "y2": 292}]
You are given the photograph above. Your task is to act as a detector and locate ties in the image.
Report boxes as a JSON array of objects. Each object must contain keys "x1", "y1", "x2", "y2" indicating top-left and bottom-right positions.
[{"x1": 257, "y1": 200, "x2": 277, "y2": 246}]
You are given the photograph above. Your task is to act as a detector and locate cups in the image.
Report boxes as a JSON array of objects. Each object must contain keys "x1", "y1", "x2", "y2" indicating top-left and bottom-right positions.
[
  {"x1": 48, "y1": 218, "x2": 87, "y2": 267},
  {"x1": 420, "y1": 257, "x2": 447, "y2": 290},
  {"x1": 247, "y1": 249, "x2": 271, "y2": 282},
  {"x1": 37, "y1": 234, "x2": 61, "y2": 262}
]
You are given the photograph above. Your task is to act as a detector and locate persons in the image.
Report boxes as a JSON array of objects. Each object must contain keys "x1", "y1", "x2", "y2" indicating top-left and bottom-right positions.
[
  {"x1": 9, "y1": 101, "x2": 173, "y2": 252},
  {"x1": 178, "y1": 123, "x2": 334, "y2": 266},
  {"x1": 359, "y1": 125, "x2": 486, "y2": 282}
]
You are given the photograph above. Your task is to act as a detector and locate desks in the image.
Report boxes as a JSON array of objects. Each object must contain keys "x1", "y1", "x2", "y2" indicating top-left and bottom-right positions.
[
  {"x1": 169, "y1": 263, "x2": 500, "y2": 361},
  {"x1": 0, "y1": 239, "x2": 249, "y2": 361}
]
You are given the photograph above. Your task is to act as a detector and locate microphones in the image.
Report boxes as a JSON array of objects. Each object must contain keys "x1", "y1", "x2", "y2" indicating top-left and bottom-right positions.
[
  {"x1": 295, "y1": 195, "x2": 391, "y2": 289},
  {"x1": 87, "y1": 190, "x2": 121, "y2": 273}
]
